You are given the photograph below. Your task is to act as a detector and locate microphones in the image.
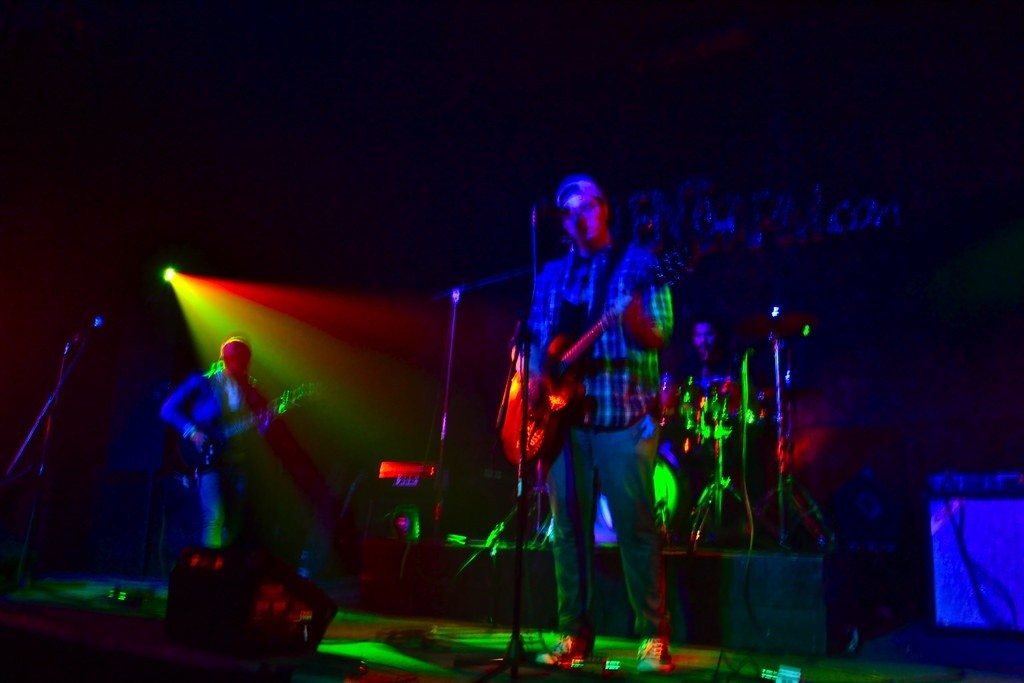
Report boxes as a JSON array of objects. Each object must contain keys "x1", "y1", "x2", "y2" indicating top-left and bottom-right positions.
[
  {"x1": 71, "y1": 317, "x2": 103, "y2": 345},
  {"x1": 539, "y1": 201, "x2": 570, "y2": 218}
]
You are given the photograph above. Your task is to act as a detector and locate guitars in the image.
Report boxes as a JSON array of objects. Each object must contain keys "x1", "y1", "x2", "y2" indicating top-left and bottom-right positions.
[
  {"x1": 495, "y1": 249, "x2": 687, "y2": 465},
  {"x1": 178, "y1": 378, "x2": 332, "y2": 466}
]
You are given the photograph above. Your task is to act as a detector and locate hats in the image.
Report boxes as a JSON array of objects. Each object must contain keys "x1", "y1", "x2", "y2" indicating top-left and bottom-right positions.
[{"x1": 555, "y1": 173, "x2": 602, "y2": 208}]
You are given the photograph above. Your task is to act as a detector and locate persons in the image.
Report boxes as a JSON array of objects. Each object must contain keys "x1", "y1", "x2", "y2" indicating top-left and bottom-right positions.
[
  {"x1": 522, "y1": 175, "x2": 675, "y2": 676},
  {"x1": 672, "y1": 317, "x2": 733, "y2": 385},
  {"x1": 159, "y1": 333, "x2": 268, "y2": 546}
]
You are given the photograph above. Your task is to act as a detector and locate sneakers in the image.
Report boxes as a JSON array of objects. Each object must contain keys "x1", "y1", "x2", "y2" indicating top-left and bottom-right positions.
[
  {"x1": 637, "y1": 634, "x2": 674, "y2": 674},
  {"x1": 536, "y1": 627, "x2": 593, "y2": 670}
]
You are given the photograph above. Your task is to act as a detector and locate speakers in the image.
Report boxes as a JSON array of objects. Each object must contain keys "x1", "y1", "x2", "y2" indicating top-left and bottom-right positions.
[
  {"x1": 167, "y1": 545, "x2": 339, "y2": 663},
  {"x1": 929, "y1": 471, "x2": 1024, "y2": 633}
]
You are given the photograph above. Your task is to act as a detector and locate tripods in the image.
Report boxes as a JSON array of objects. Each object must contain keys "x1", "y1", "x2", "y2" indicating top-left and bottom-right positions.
[
  {"x1": 474, "y1": 219, "x2": 624, "y2": 683},
  {"x1": 688, "y1": 337, "x2": 837, "y2": 554}
]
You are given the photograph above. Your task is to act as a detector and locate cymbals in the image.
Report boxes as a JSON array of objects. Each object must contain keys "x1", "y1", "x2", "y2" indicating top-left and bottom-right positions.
[{"x1": 736, "y1": 309, "x2": 816, "y2": 337}]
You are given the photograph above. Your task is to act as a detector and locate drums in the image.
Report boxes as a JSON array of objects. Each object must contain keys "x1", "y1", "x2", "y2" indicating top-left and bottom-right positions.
[{"x1": 642, "y1": 439, "x2": 691, "y2": 530}]
